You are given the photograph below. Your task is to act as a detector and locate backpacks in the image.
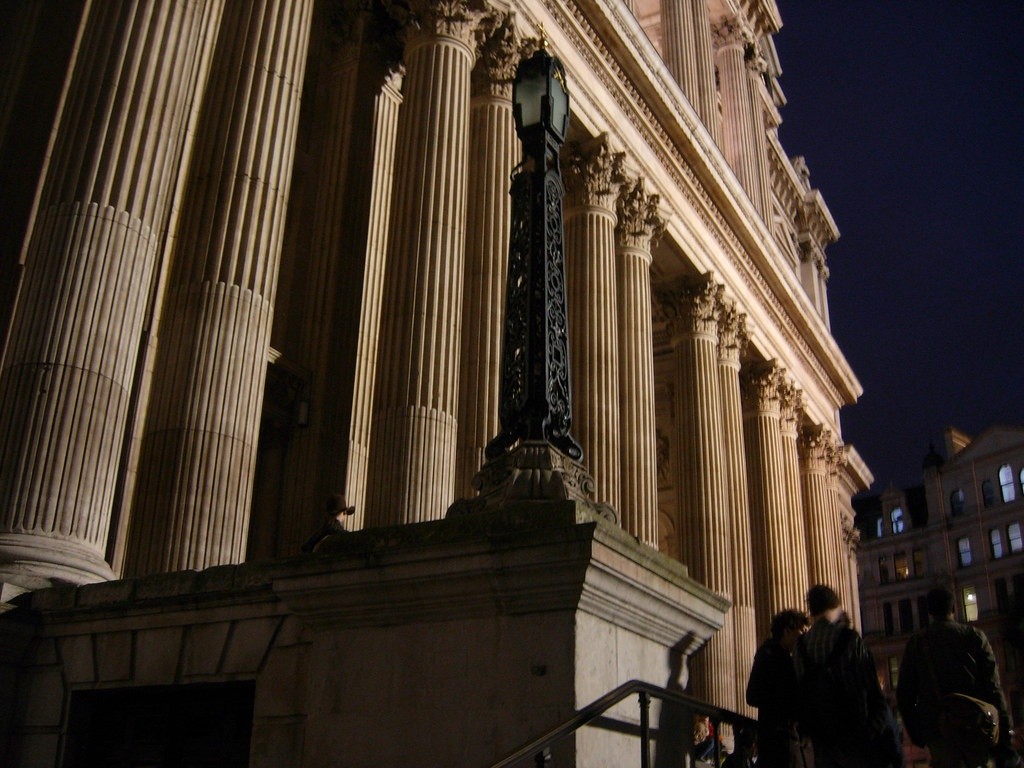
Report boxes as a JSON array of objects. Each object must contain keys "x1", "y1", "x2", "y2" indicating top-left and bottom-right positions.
[{"x1": 797, "y1": 629, "x2": 853, "y2": 737}]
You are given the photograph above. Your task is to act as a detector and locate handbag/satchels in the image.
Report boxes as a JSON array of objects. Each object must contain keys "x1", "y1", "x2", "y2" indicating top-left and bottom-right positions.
[{"x1": 937, "y1": 692, "x2": 1000, "y2": 749}]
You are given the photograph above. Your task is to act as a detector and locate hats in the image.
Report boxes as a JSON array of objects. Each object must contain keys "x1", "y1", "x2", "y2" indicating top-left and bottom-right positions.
[{"x1": 809, "y1": 585, "x2": 838, "y2": 616}]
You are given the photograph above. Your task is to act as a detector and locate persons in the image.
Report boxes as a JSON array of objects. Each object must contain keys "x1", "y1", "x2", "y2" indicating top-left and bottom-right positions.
[
  {"x1": 746, "y1": 586, "x2": 905, "y2": 768},
  {"x1": 694, "y1": 716, "x2": 758, "y2": 768},
  {"x1": 896, "y1": 591, "x2": 1014, "y2": 768}
]
